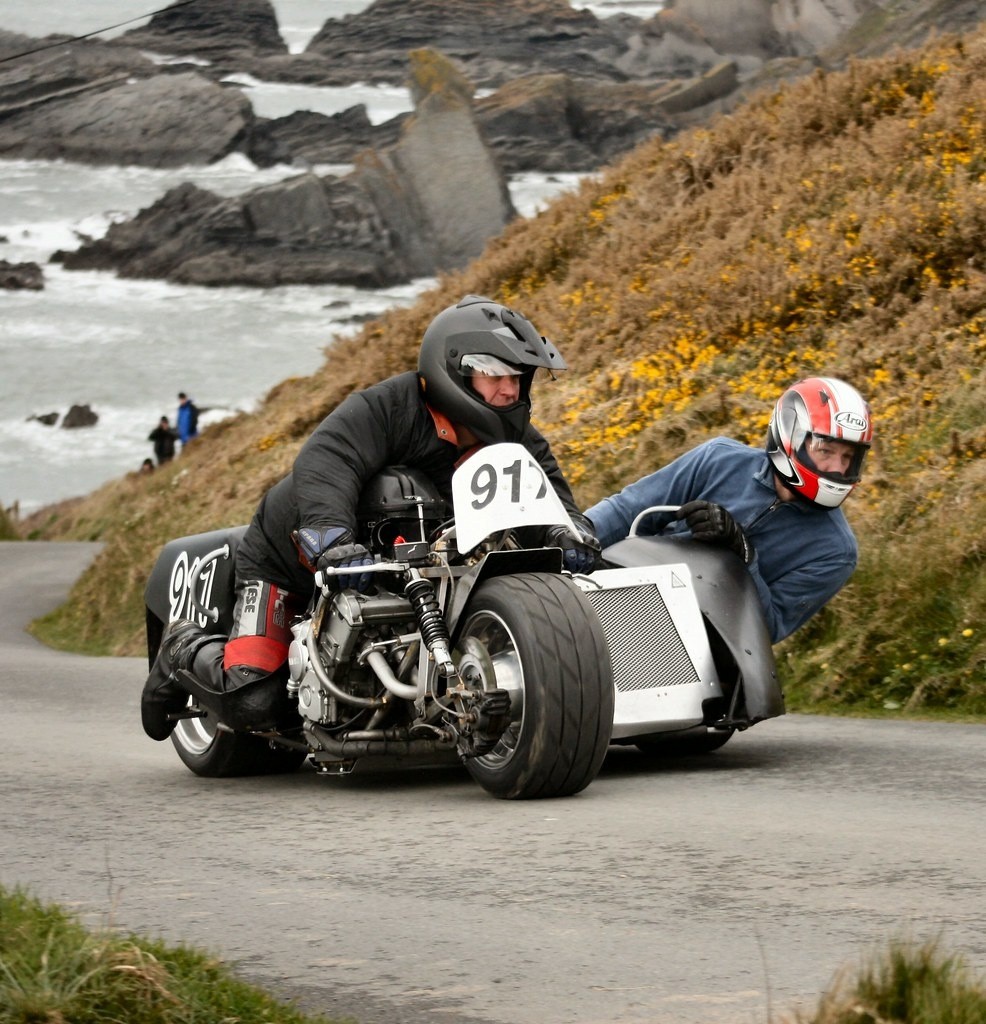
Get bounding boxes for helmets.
[767,375,871,508]
[416,295,569,444]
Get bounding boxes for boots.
[142,618,226,740]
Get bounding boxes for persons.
[141,458,154,473]
[147,416,178,467]
[174,392,199,452]
[138,292,602,743]
[581,374,874,647]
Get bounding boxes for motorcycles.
[146,437,791,798]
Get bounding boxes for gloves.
[674,499,756,567]
[314,541,376,592]
[548,525,604,575]
